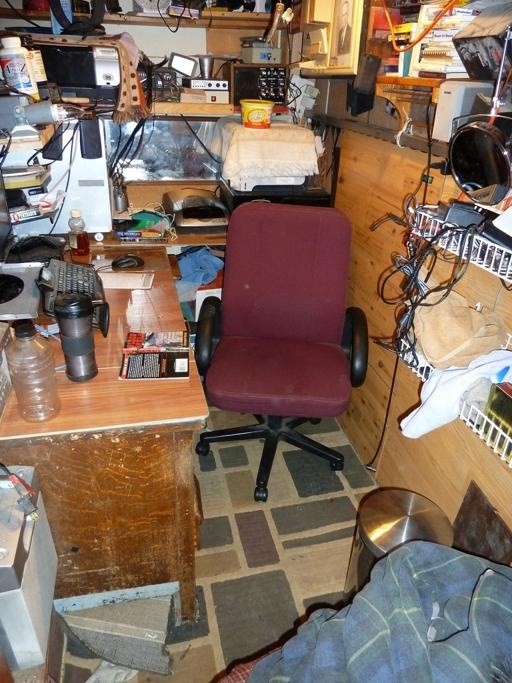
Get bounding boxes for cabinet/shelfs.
[0,7,299,120]
[396,203,512,473]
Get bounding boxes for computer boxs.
[0,117,114,236]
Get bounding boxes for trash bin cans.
[343,487,455,606]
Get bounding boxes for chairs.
[192,200,372,501]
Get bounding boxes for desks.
[0,222,226,628]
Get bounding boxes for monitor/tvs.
[0,164,24,305]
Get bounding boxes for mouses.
[111,253,145,269]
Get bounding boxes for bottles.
[68,219,91,264]
[6,321,61,424]
[0,34,47,95]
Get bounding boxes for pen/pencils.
[138,332,154,350]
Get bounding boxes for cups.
[54,292,110,382]
[199,58,214,78]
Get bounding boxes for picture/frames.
[299,0,371,79]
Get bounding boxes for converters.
[435,203,484,229]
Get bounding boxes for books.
[9,204,43,224]
[365,0,484,80]
[117,330,190,382]
[114,221,169,244]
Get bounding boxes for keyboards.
[39,257,106,318]
